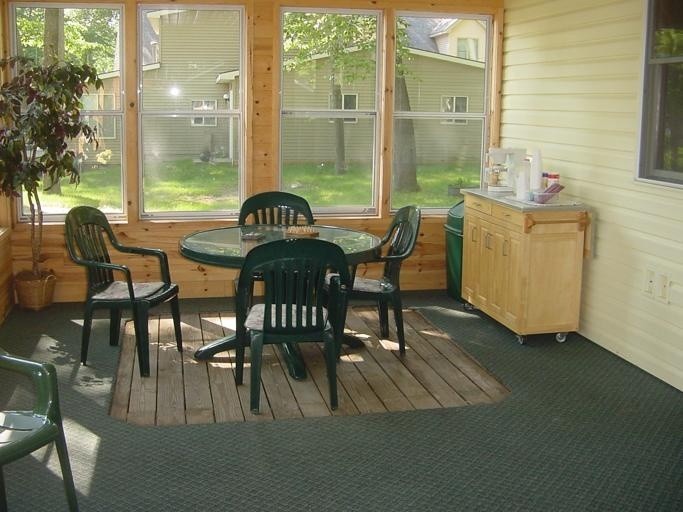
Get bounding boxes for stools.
[0,349,82,511]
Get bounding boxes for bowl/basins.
[533,192,559,206]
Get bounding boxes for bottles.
[541,172,547,190]
[514,158,532,200]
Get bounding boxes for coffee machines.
[484,148,526,192]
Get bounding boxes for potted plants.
[0,41,106,314]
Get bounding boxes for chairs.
[325,205,421,356]
[237,192,315,228]
[65,205,185,378]
[234,239,353,414]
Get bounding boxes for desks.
[177,227,381,365]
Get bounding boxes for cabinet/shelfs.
[461,187,591,346]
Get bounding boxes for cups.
[547,174,559,188]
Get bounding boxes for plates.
[240,233,264,240]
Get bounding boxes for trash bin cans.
[443,200,464,300]
